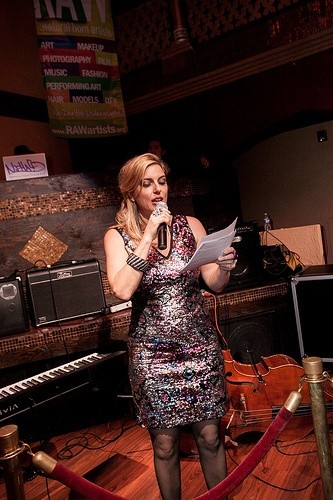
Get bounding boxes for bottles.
[264,213,271,231]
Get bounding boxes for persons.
[104,153,238,500]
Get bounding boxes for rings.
[153,209,163,216]
[233,253,237,258]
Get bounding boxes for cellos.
[176,347,333,449]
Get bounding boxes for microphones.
[155,201,168,248]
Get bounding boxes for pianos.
[0,347,138,445]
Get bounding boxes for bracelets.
[126,253,150,272]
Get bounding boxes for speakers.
[26,260,107,327]
[0,275,30,339]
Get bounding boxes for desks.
[0,281,290,384]
